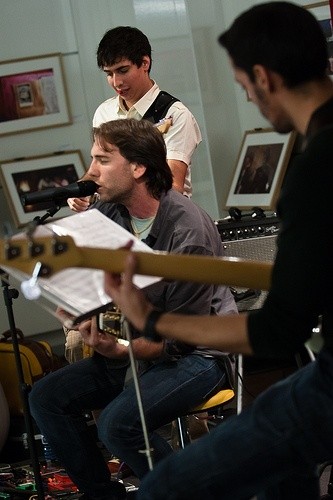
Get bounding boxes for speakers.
[213,214,282,311]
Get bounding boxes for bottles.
[42,435,59,468]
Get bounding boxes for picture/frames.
[300,0,333,83]
[0,51,73,137]
[0,148,88,228]
[220,127,297,210]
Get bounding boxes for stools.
[172,389,235,449]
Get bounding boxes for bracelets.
[143,307,169,343]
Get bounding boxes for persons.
[38,26,202,478]
[105,0,333,500]
[28,118,239,500]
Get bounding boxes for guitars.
[62,307,131,367]
[0,233,273,300]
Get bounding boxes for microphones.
[233,287,263,302]
[19,180,98,206]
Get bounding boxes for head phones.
[228,206,265,221]
